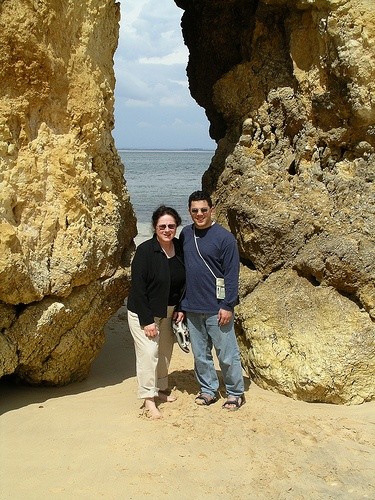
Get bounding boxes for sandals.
[195,393,219,405]
[222,394,245,410]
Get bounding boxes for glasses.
[192,207,207,213]
[159,224,177,230]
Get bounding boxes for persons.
[179,191,246,410]
[127,205,185,420]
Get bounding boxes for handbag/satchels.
[216,278,225,299]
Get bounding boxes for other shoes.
[172,320,190,354]
